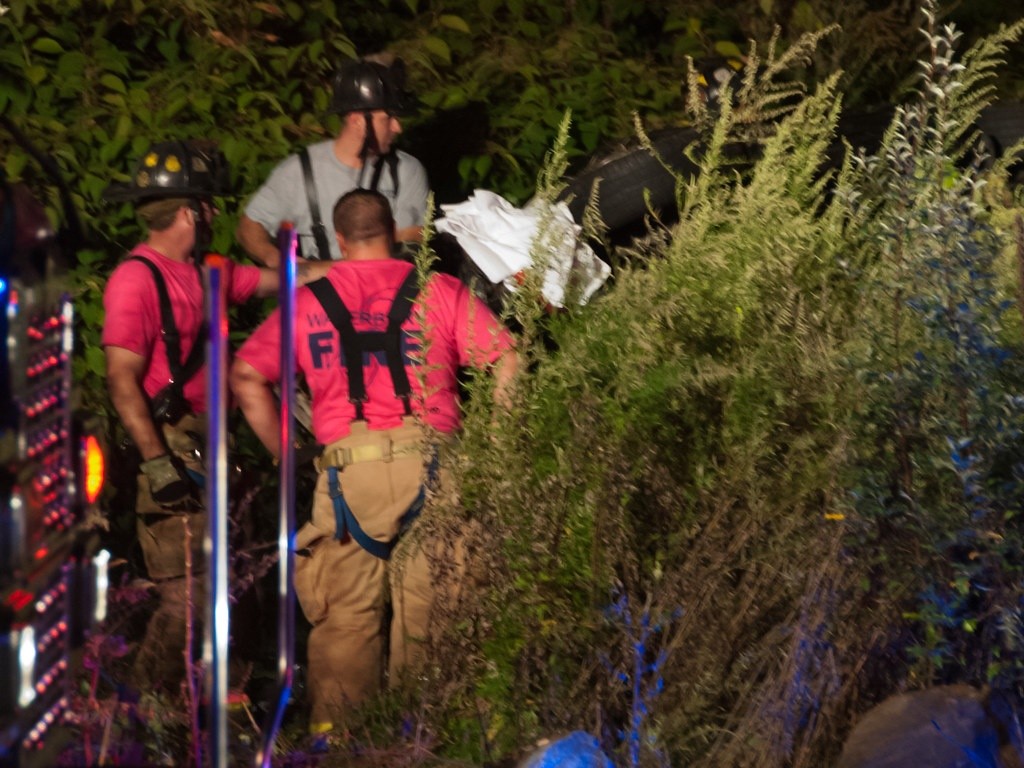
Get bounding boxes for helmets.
[336,60,411,112]
[127,140,232,200]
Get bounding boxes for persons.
[102,62,521,754]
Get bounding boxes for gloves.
[295,444,324,495]
[138,449,206,514]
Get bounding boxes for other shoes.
[179,680,251,713]
[312,727,350,746]
